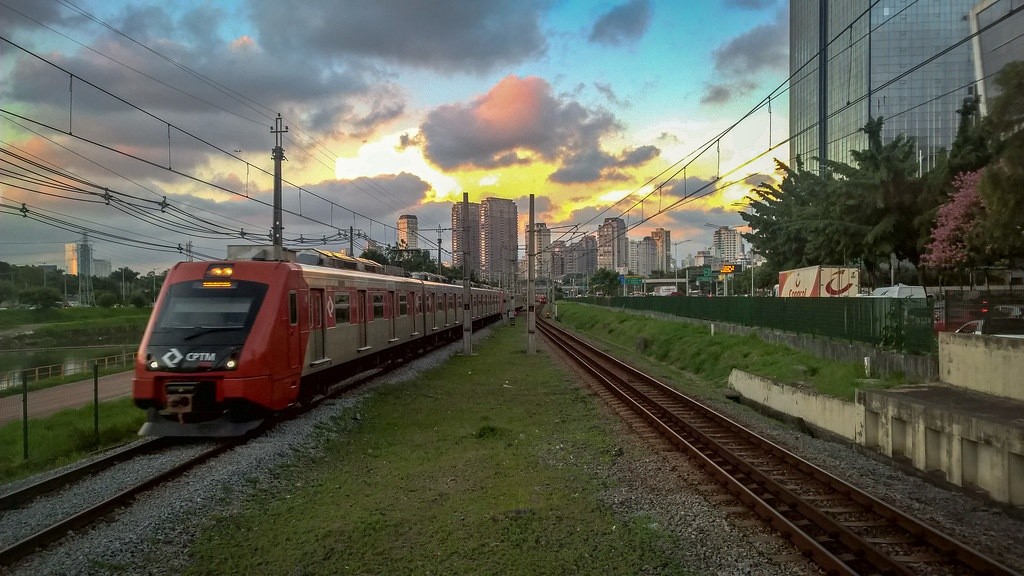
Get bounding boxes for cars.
[995,304,1024,318]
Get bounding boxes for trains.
[132,259,505,441]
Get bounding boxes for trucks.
[765,264,859,297]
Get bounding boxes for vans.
[869,286,927,299]
